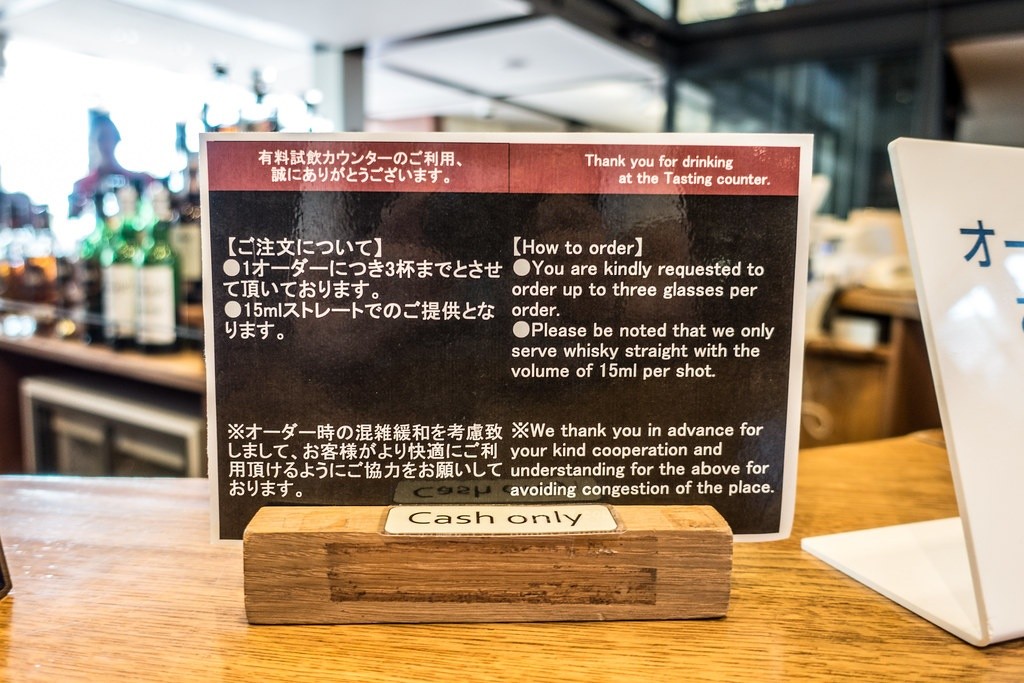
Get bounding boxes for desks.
[0,431,1024,683]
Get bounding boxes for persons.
[67,115,156,224]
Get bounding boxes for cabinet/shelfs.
[798,289,943,451]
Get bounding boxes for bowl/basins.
[832,318,879,351]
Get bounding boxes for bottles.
[134,189,184,352]
[102,189,138,350]
[81,191,113,341]
